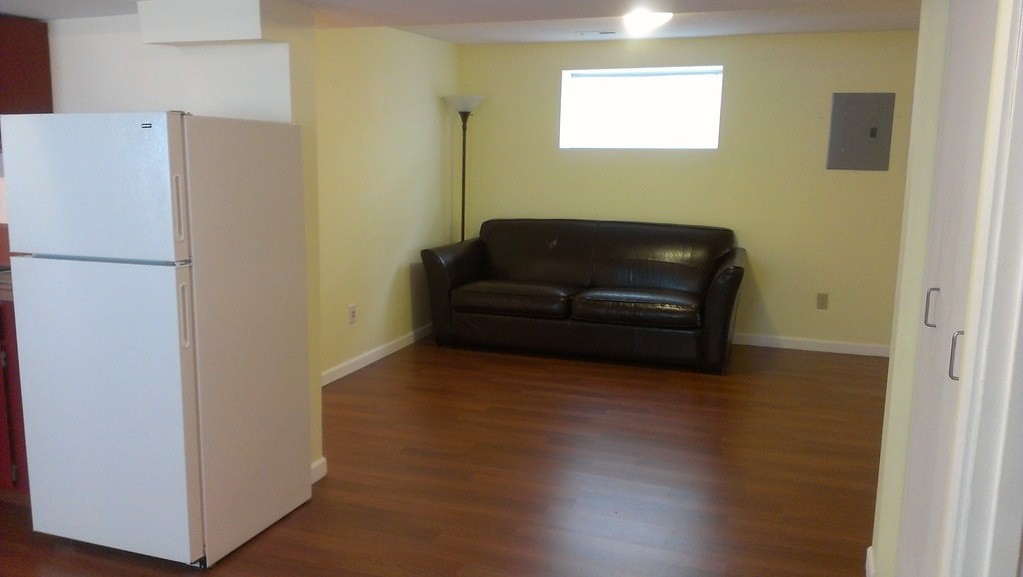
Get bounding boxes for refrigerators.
[0,110,312,570]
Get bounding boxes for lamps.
[442,95,483,241]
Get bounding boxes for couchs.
[421,218,748,374]
